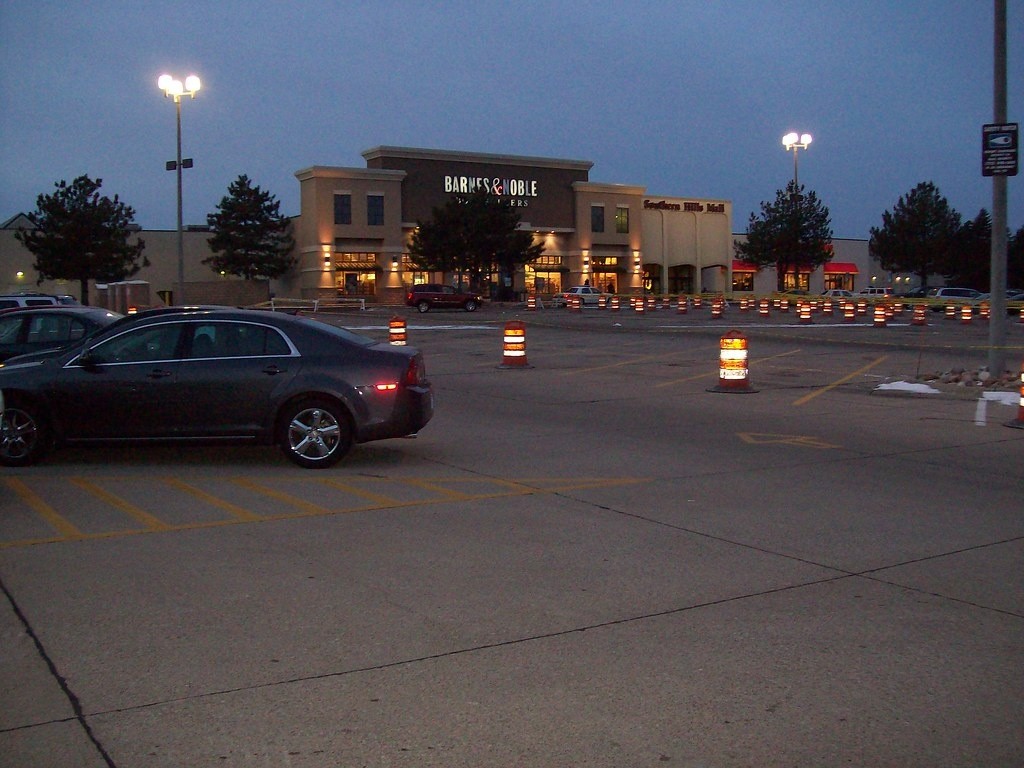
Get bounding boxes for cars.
[0,307,159,365]
[820,290,854,302]
[973,290,1024,316]
[556,285,614,307]
[777,290,820,306]
[0,305,434,471]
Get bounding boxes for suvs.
[0,294,65,328]
[926,288,982,313]
[852,287,897,299]
[59,296,84,310]
[405,284,484,312]
[896,286,944,311]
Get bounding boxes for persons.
[607,283,615,293]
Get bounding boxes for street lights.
[781,131,812,289]
[157,74,201,305]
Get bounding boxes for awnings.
[825,263,859,274]
[592,265,626,273]
[776,262,814,273]
[529,264,570,272]
[336,262,382,271]
[732,260,762,272]
[404,263,438,271]
